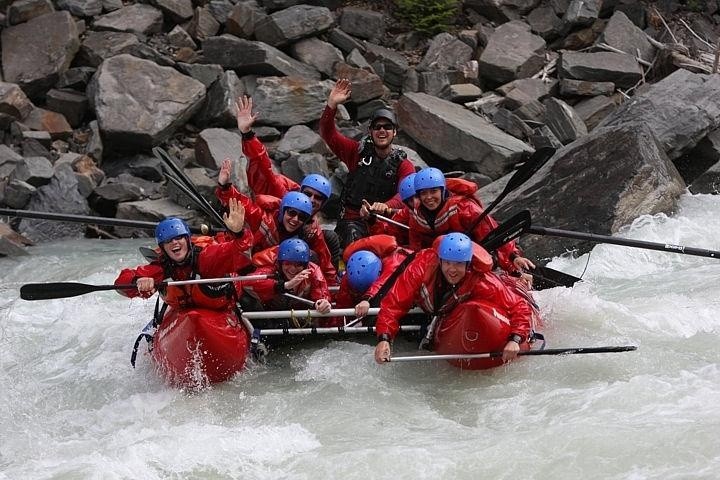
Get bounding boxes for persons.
[408,166,536,272]
[369,172,532,291]
[214,157,320,266]
[115,197,254,310]
[242,237,332,315]
[234,94,339,287]
[330,234,414,329]
[320,77,416,250]
[374,231,534,363]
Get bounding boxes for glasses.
[372,124,394,130]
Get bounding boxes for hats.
[371,109,397,127]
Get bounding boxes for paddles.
[384,345,636,361]
[152,146,228,230]
[462,146,555,235]
[20,274,280,300]
[368,210,581,287]
[479,210,532,251]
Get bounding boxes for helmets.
[437,232,473,263]
[155,217,191,245]
[346,250,382,291]
[398,167,446,202]
[278,173,333,266]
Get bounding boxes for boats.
[144,265,533,394]
